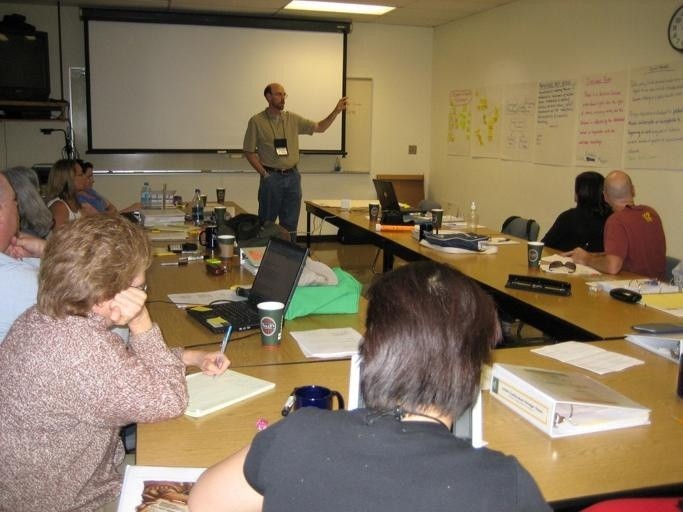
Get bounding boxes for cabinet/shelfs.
[373,173,425,210]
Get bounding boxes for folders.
[489,363,652,438]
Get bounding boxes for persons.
[539,171,613,253]
[241,83,349,239]
[560,171,666,282]
[1,159,232,512]
[187,261,553,512]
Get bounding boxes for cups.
[527,240,545,268]
[295,385,345,413]
[367,203,380,223]
[419,208,444,239]
[199,188,235,261]
[257,301,285,349]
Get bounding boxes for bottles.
[140,182,152,208]
[467,202,479,234]
[191,188,204,225]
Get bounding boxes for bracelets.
[263,171,267,176]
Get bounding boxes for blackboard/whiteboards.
[70,67,374,174]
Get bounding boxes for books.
[184,367,276,419]
[632,324,683,335]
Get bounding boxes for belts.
[263,164,297,175]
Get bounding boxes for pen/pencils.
[160,250,210,265]
[282,388,299,416]
[497,239,510,242]
[584,243,588,249]
[212,326,232,380]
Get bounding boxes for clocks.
[667,4,683,50]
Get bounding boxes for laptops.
[186,236,310,335]
[373,179,428,216]
